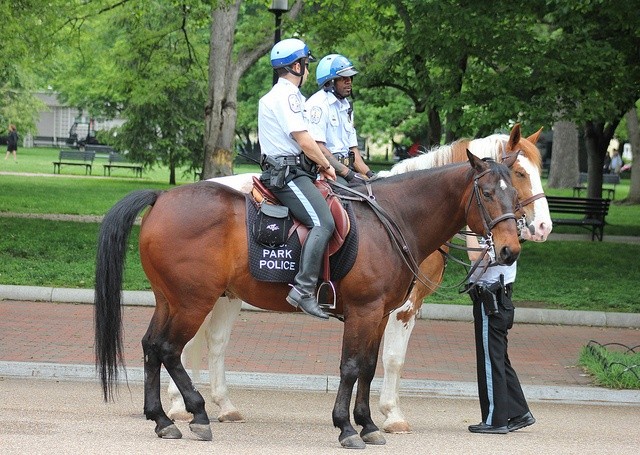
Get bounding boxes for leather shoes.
[508,412,535,432]
[468,422,508,434]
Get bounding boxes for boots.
[285,226,329,321]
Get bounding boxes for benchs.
[572,173,620,200]
[545,195,611,243]
[53,149,96,175]
[56,136,79,149]
[103,153,143,178]
[33,136,56,148]
[83,145,114,165]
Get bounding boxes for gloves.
[350,173,366,185]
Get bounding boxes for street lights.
[268,0,292,87]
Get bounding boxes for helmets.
[316,54,359,90]
[271,39,317,69]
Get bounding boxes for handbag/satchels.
[256,202,288,246]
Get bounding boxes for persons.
[602,151,612,174]
[609,148,622,182]
[465,222,536,434]
[4,124,19,163]
[257,38,338,320]
[305,53,377,186]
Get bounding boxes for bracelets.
[323,163,331,171]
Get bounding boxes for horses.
[92,147,522,449]
[165,122,552,437]
